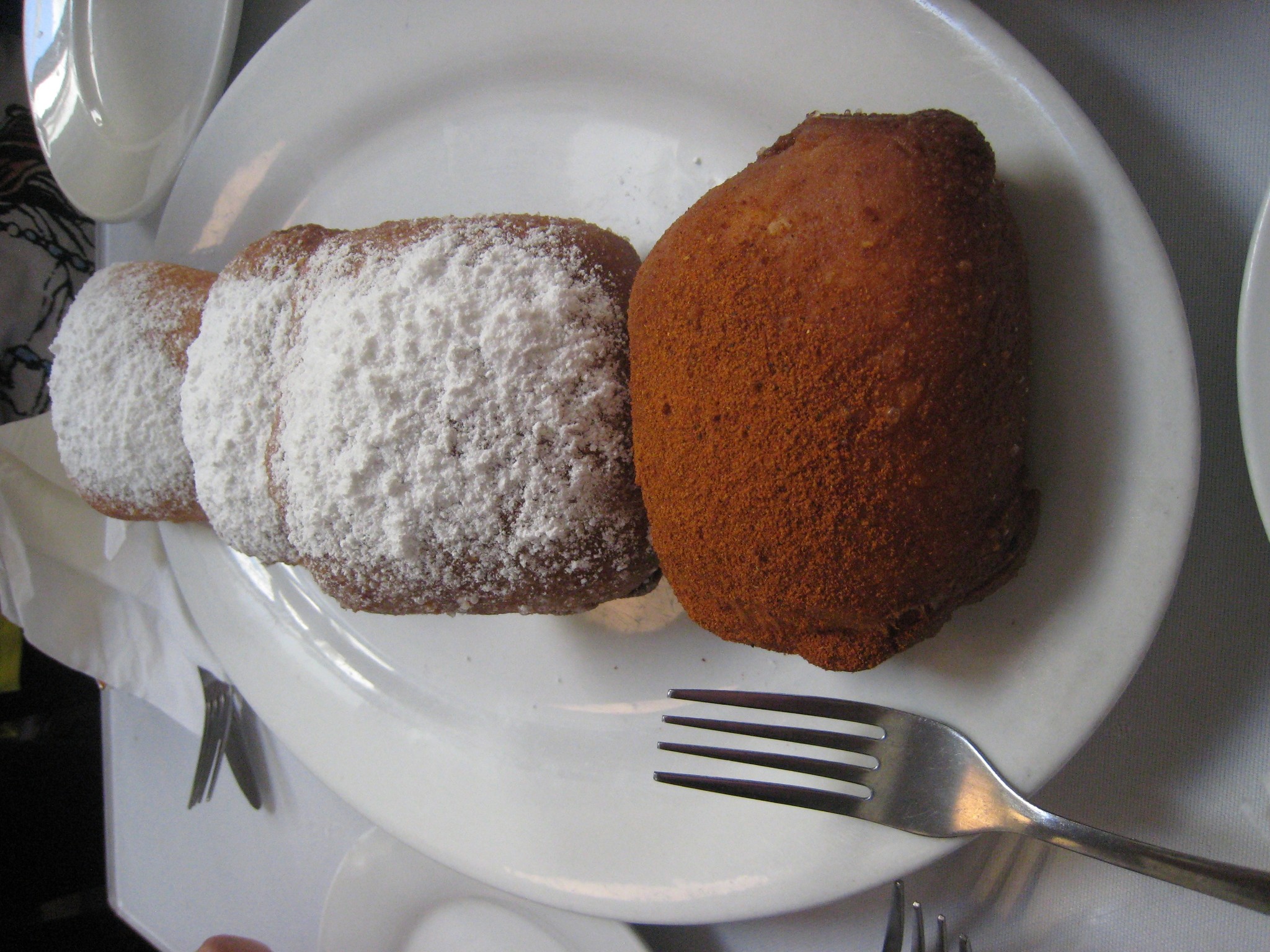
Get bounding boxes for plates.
[317,827,651,952]
[1236,186,1269,544]
[21,0,240,224]
[153,2,1205,926]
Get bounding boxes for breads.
[282,209,661,626]
[42,260,216,526]
[607,108,1040,678]
[172,224,346,571]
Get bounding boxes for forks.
[186,663,229,808]
[881,878,974,952]
[654,685,1270,915]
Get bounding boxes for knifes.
[224,700,260,811]
